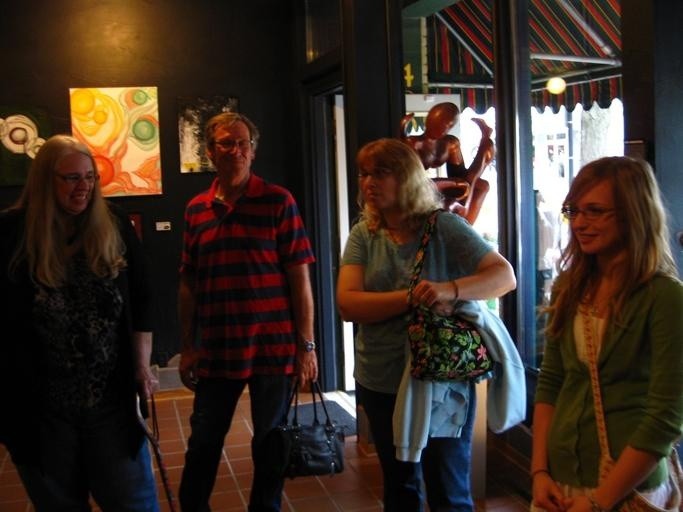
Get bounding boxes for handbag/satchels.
[598,449,683,512]
[405,308,493,381]
[274,419,343,477]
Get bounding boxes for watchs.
[587,493,610,512]
[297,340,315,352]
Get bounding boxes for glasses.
[56,170,96,183]
[562,205,618,220]
[356,169,401,180]
[212,140,255,151]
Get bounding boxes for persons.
[397,102,494,226]
[336,139,517,512]
[534,201,555,357]
[176,111,316,512]
[0,135,161,512]
[529,157,683,512]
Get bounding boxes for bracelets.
[530,470,552,484]
[448,279,459,306]
